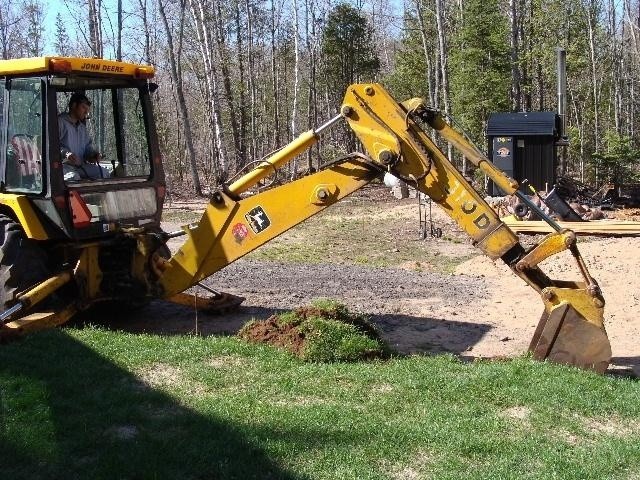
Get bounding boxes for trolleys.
[416,185,442,240]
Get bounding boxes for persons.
[54,93,113,184]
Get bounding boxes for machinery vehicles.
[1,54,614,381]
